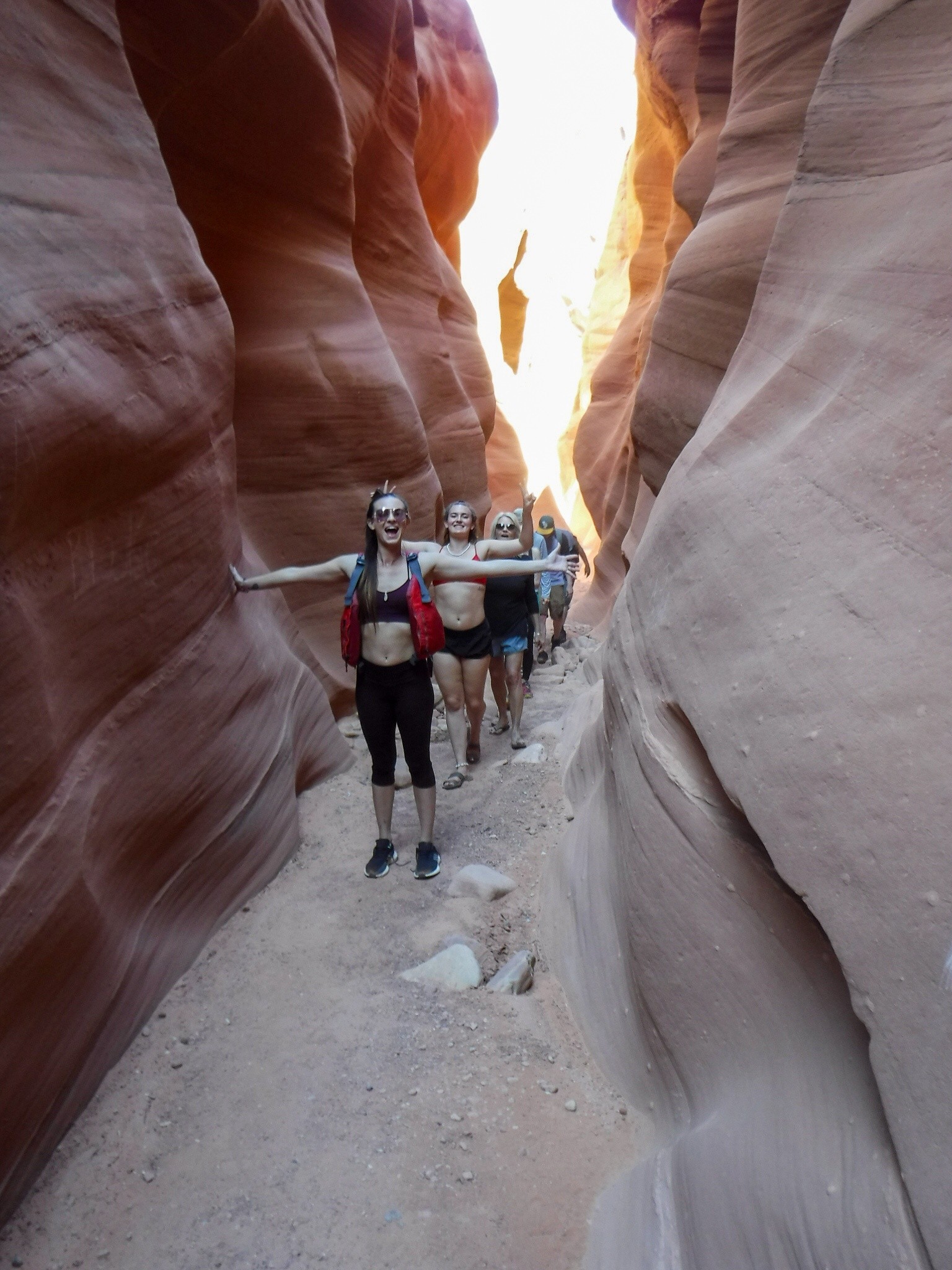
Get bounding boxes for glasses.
[496,524,515,530]
[371,508,406,521]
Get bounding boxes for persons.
[230,493,582,881]
[370,477,537,789]
[483,508,590,749]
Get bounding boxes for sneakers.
[411,841,440,878]
[364,839,411,878]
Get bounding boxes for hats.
[538,515,554,535]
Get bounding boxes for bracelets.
[242,578,248,592]
[535,632,541,636]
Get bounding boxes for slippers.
[443,772,468,789]
[489,725,509,734]
[467,742,480,763]
[511,737,526,749]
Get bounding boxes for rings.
[564,568,568,573]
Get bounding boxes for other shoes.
[523,681,532,698]
[560,630,566,643]
[551,634,560,652]
[537,651,548,664]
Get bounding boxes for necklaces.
[447,542,470,556]
